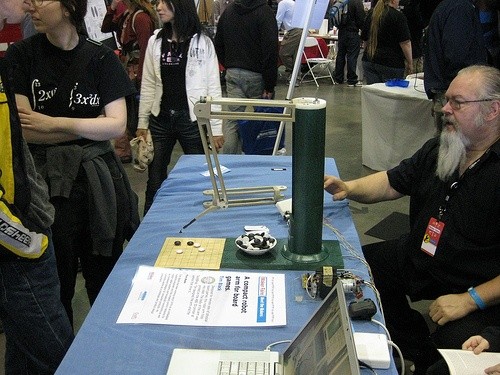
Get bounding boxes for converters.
[354,332,390,369]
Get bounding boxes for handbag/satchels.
[236,98,285,155]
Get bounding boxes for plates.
[235,233,277,256]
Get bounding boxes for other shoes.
[286,72,302,81]
[347,80,364,87]
[121,155,133,163]
[287,81,299,86]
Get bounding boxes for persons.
[362,0,415,84]
[136,0,225,217]
[423,0,495,137]
[215,0,281,155]
[329,0,367,87]
[0,1,163,375]
[325,64,500,375]
[275,0,303,87]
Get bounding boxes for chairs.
[300,37,335,88]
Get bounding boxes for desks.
[360,76,437,171]
[54,156,399,375]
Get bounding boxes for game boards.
[152,237,227,271]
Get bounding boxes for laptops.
[167,280,361,375]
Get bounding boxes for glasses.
[24,0,59,7]
[439,94,492,110]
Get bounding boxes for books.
[437,349,500,375]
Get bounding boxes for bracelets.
[468,287,486,309]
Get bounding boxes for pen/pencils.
[272,168,287,171]
